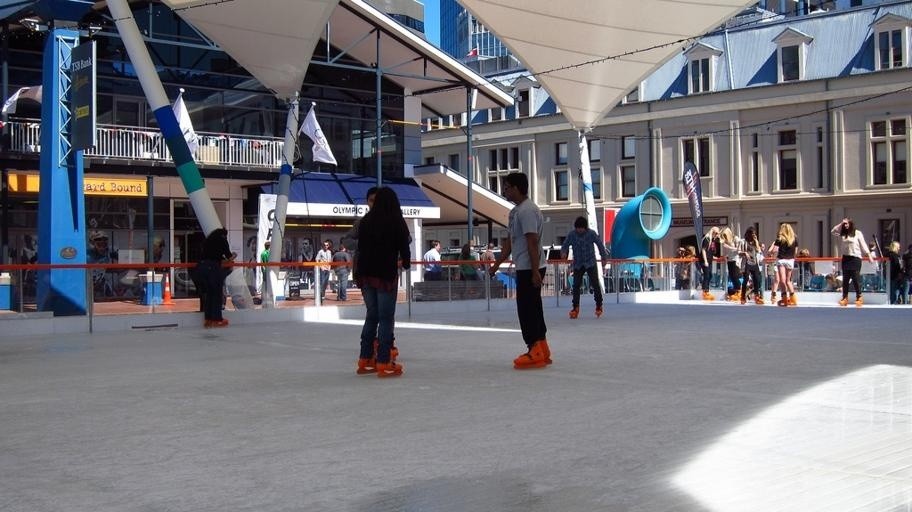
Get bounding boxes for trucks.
[438,244,516,274]
[540,244,573,284]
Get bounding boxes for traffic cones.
[162,272,178,308]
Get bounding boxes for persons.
[145,236,170,272]
[261,242,272,279]
[561,216,607,315]
[86,231,118,297]
[298,237,316,271]
[317,242,498,303]
[674,216,912,307]
[23,235,38,282]
[199,229,237,326]
[244,236,257,286]
[489,173,550,364]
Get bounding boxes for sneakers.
[356,355,376,368]
[202,317,228,327]
[700,289,865,307]
[567,304,605,318]
[537,338,552,359]
[375,361,403,372]
[513,343,545,365]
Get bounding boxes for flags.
[173,96,199,161]
[301,108,338,166]
[466,48,477,57]
